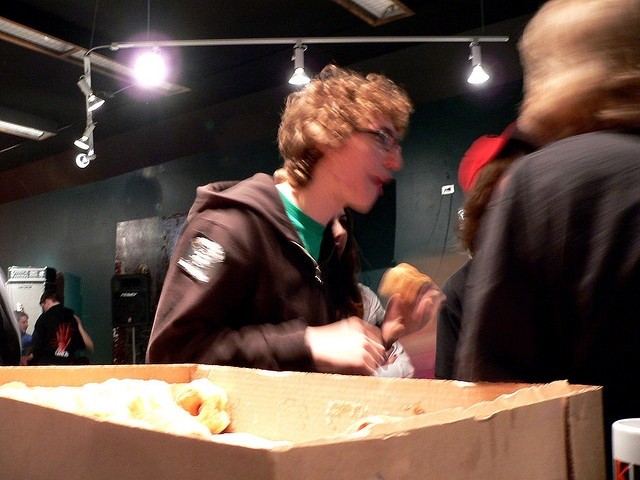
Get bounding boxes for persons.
[437,113,537,380]
[434,0,640,480]
[145,64,445,377]
[31,291,94,365]
[14,310,32,356]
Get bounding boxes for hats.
[458,123,517,194]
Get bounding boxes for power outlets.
[441,184,454,196]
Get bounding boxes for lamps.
[466,45,491,84]
[77,74,105,112]
[74,123,96,151]
[76,153,96,168]
[288,45,313,85]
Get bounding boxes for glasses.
[353,128,403,152]
